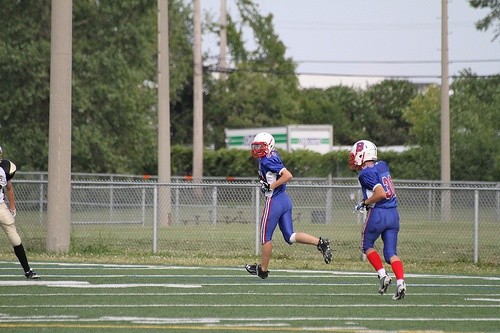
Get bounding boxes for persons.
[0,146,41,279]
[245,132,332,279]
[348,140,406,300]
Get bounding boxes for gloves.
[353,199,366,214]
[10,209,16,217]
[258,176,271,192]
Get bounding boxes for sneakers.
[244,263,268,280]
[24,269,41,281]
[376,270,393,293]
[391,281,406,301]
[316,236,333,265]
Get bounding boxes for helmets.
[251,132,275,159]
[348,139,378,171]
